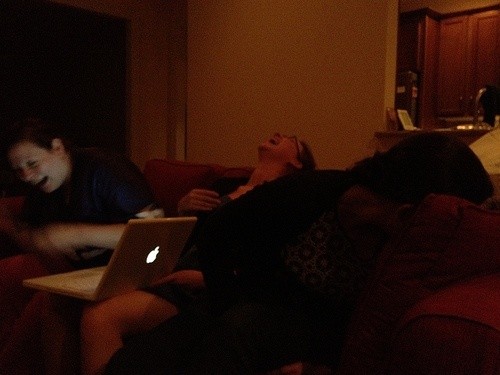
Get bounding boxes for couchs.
[0,158,500,375]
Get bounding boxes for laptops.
[22,216,197,302]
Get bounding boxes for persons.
[103,132,500,375]
[0,115,165,374]
[39,131,317,375]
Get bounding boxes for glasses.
[287,135,300,160]
